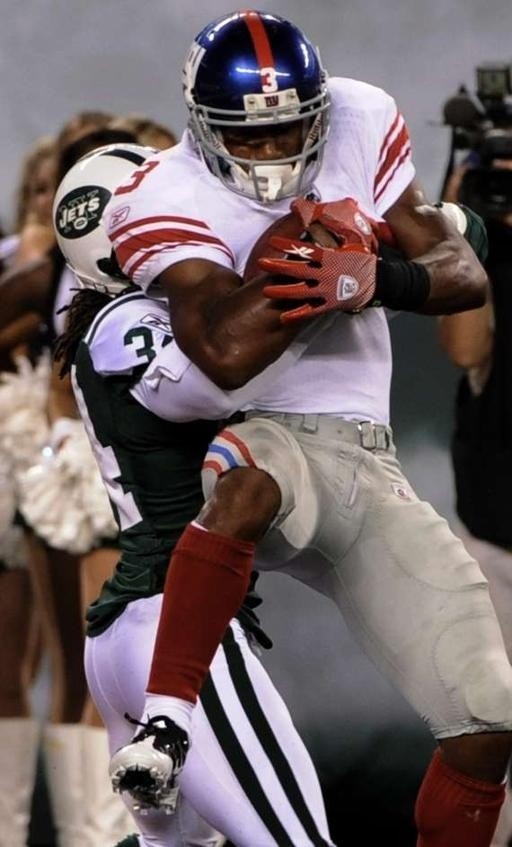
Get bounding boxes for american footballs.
[244,212,315,287]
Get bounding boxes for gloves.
[259,194,380,326]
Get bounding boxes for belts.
[271,411,390,451]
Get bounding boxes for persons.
[1,110,332,844]
[99,9,511,847]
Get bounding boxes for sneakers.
[109,718,187,815]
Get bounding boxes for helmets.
[50,144,165,299]
[180,10,331,202]
